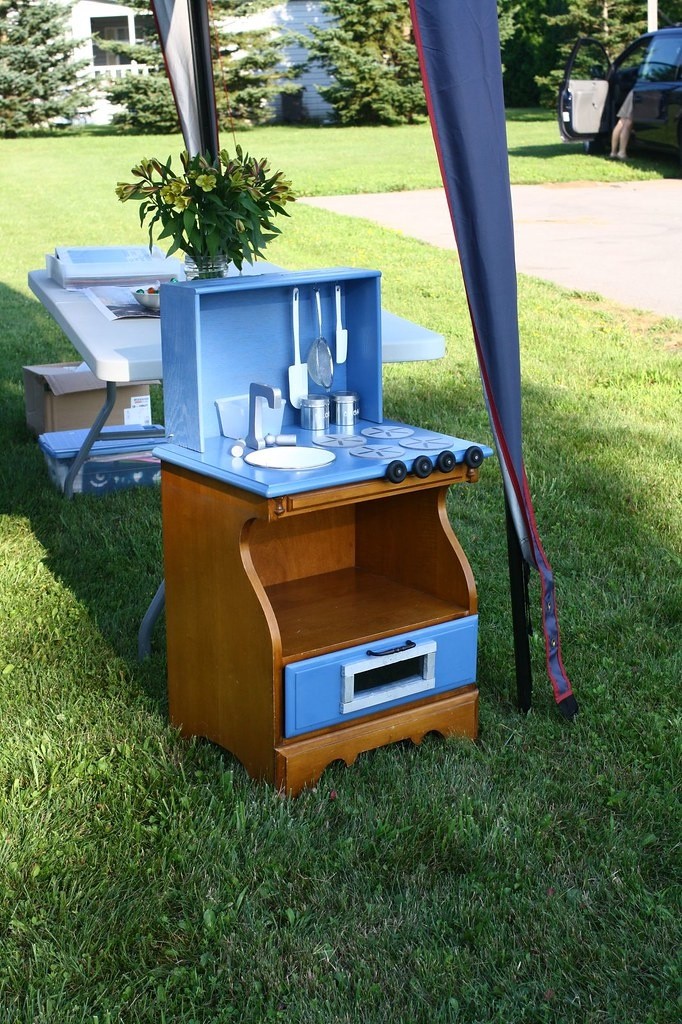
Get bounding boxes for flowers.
[115,144,298,280]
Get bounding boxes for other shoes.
[610,153,617,158]
[617,153,628,159]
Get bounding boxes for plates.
[131,290,159,307]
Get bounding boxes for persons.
[610,88,633,159]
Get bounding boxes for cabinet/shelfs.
[162,458,479,803]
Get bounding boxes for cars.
[557,27,682,160]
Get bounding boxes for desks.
[27,256,445,658]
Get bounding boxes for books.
[54,244,178,289]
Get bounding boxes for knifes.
[335,286,348,364]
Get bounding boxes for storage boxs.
[23,362,153,443]
[215,393,287,439]
[38,424,165,496]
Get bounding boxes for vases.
[184,255,228,279]
